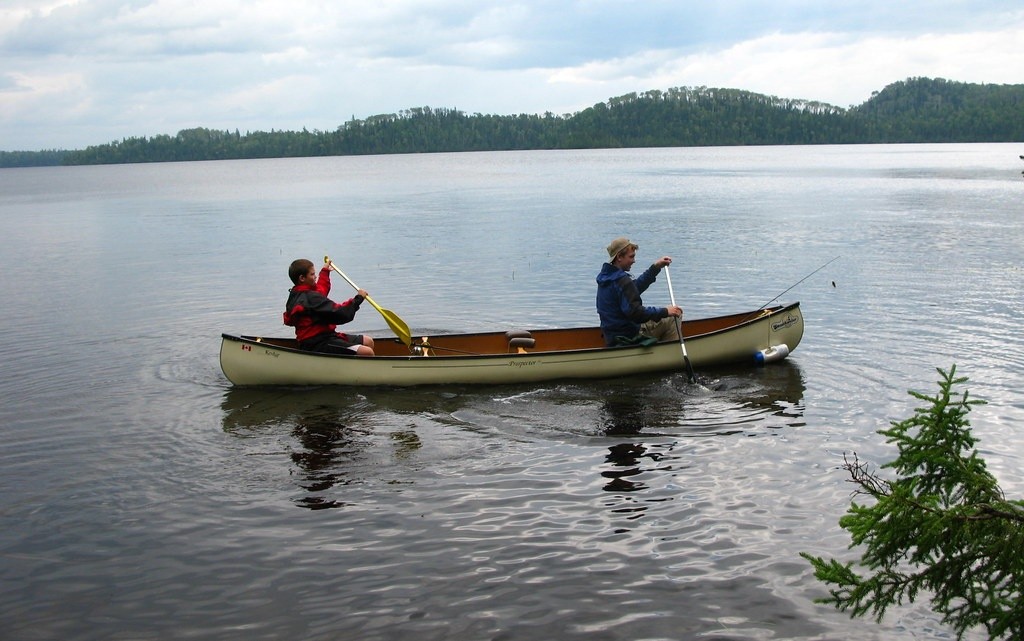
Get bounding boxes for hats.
[606,237,631,264]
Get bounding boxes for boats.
[218,299,806,388]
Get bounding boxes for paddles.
[325,257,411,348]
[664,264,697,385]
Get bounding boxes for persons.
[283,258,375,356]
[596,236,683,347]
